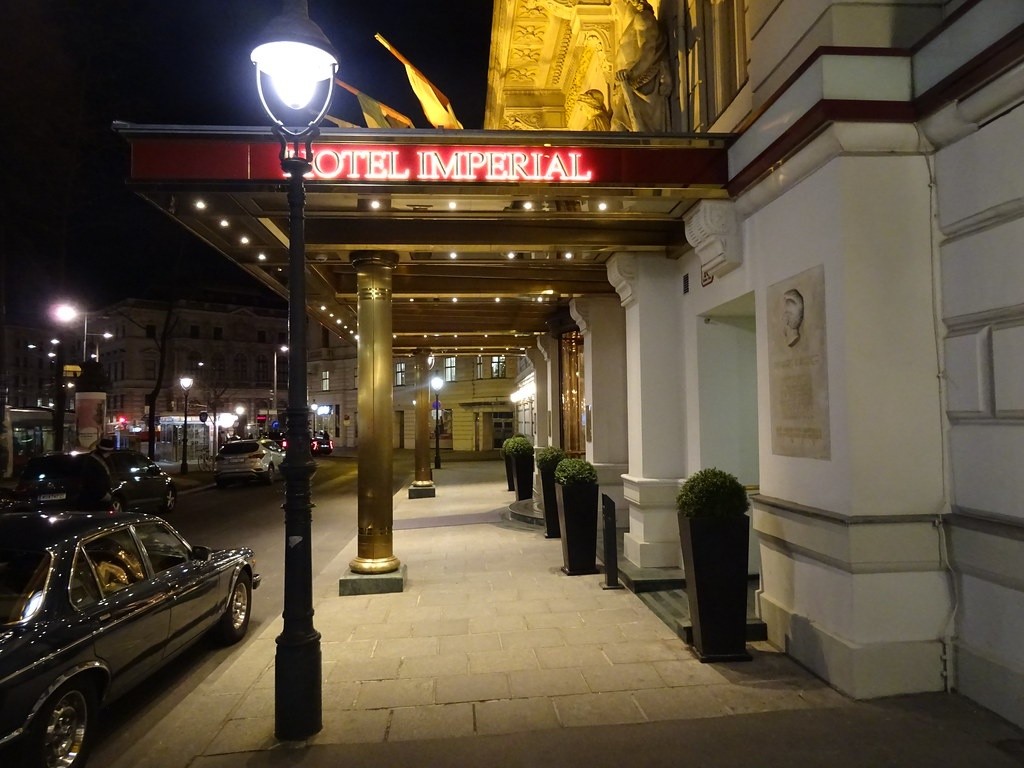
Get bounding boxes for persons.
[610,0,663,132]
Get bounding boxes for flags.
[407,64,463,131]
[356,93,415,129]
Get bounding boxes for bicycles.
[194,448,214,472]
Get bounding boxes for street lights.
[248,1,342,740]
[82,312,114,362]
[430,376,446,469]
[49,301,79,452]
[179,369,193,474]
[310,403,319,437]
[274,346,290,409]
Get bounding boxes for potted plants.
[554,459,599,576]
[502,433,526,492]
[674,467,754,664]
[508,436,534,502]
[536,445,568,539]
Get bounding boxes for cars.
[0,450,178,519]
[268,429,334,459]
[0,513,262,768]
[213,439,286,485]
